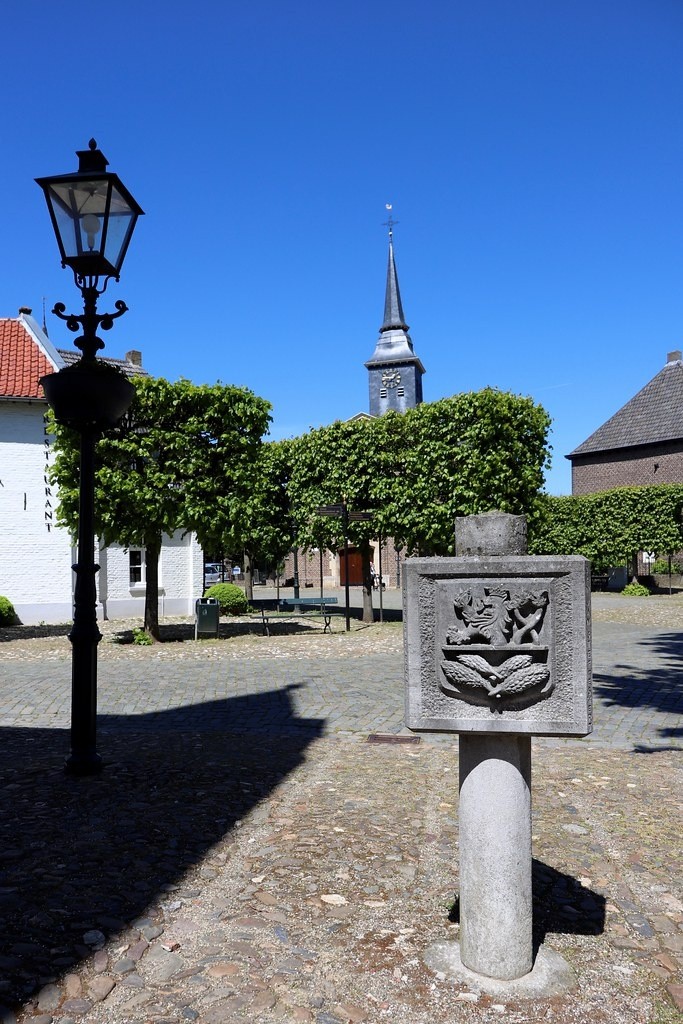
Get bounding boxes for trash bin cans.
[608,567,628,589]
[194,598,220,640]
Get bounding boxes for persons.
[370,562,376,585]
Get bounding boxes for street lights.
[33,136,145,784]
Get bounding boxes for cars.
[204,567,221,588]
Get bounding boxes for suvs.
[205,563,230,582]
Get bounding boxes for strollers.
[373,574,385,592]
